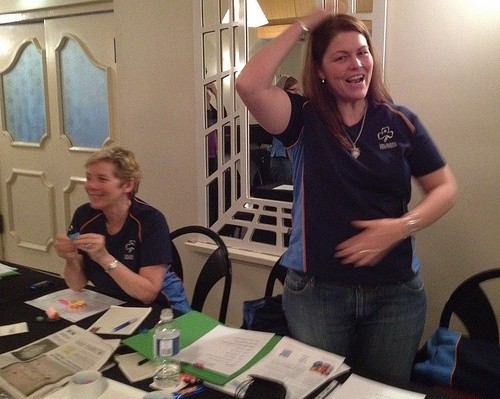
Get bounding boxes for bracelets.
[401,213,418,237]
[295,18,309,42]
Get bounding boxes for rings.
[87,244,93,249]
[359,250,367,258]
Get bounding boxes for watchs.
[105,259,118,274]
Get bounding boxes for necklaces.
[335,98,367,159]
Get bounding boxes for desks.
[0,256,455,399]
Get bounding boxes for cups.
[69,370,102,399]
[141,391,175,399]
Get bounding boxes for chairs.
[437,266,500,398]
[169,225,233,324]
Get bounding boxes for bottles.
[152,308,180,389]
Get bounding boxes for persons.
[234,9,457,391]
[269,75,300,183]
[52,145,173,309]
[18,339,57,359]
[206,68,231,219]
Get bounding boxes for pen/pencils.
[113,318,139,333]
[137,358,149,366]
